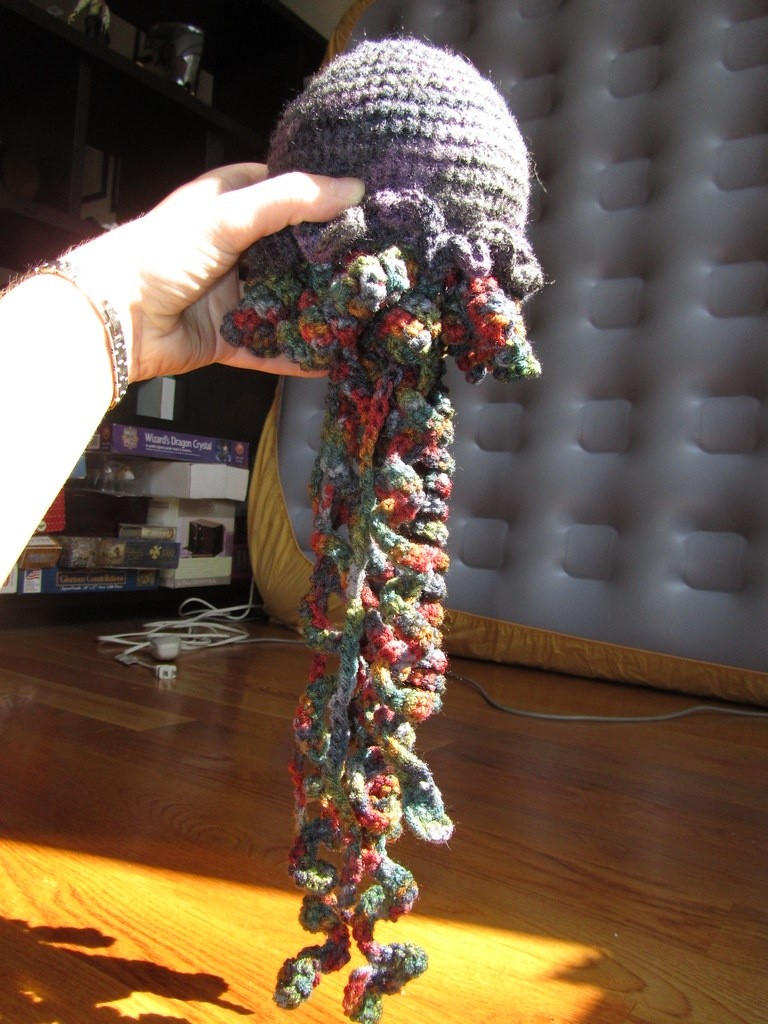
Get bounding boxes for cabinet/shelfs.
[0,0,326,627]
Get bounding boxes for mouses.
[150,636,181,661]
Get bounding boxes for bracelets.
[34,260,129,409]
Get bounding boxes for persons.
[0,163,366,590]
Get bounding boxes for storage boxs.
[135,459,250,502]
[99,423,249,468]
[17,535,63,567]
[18,567,159,595]
[57,535,180,571]
[147,496,236,589]
[0,563,18,594]
